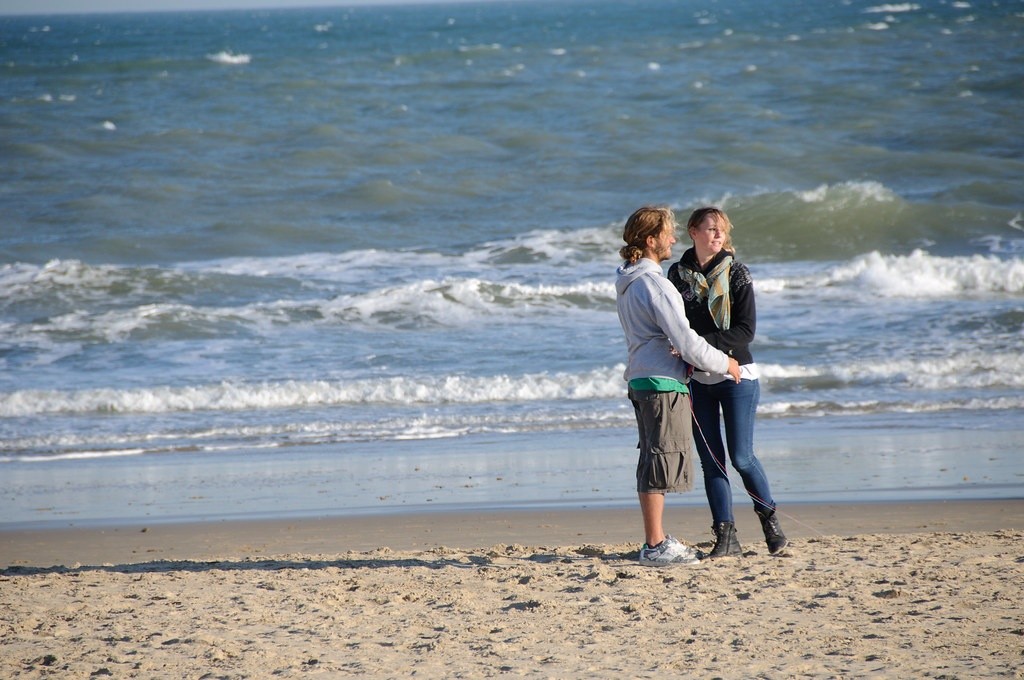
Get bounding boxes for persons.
[668,207,789,562]
[616,204,741,567]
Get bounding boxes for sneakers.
[639,534,703,566]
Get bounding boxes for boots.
[754,503,788,555]
[699,519,744,560]
